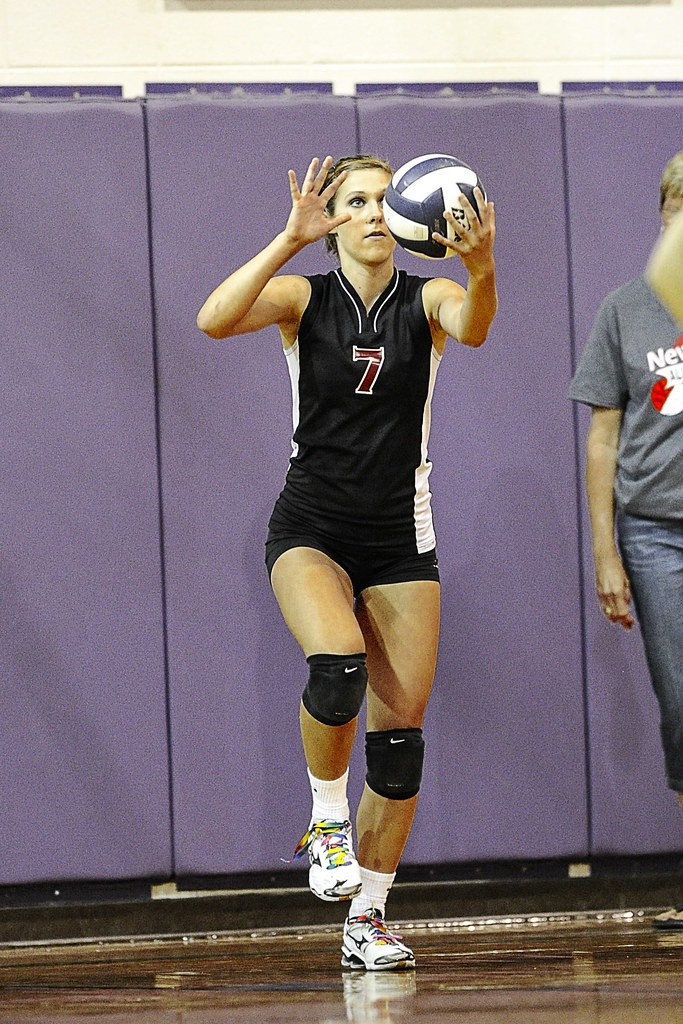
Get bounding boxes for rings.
[603,606,610,616]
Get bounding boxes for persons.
[570,148,683,807]
[196,154,499,967]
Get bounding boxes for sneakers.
[340,907,415,970]
[289,818,362,901]
[344,972,417,1019]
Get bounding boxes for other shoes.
[651,903,683,928]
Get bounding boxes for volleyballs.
[381,152,490,262]
